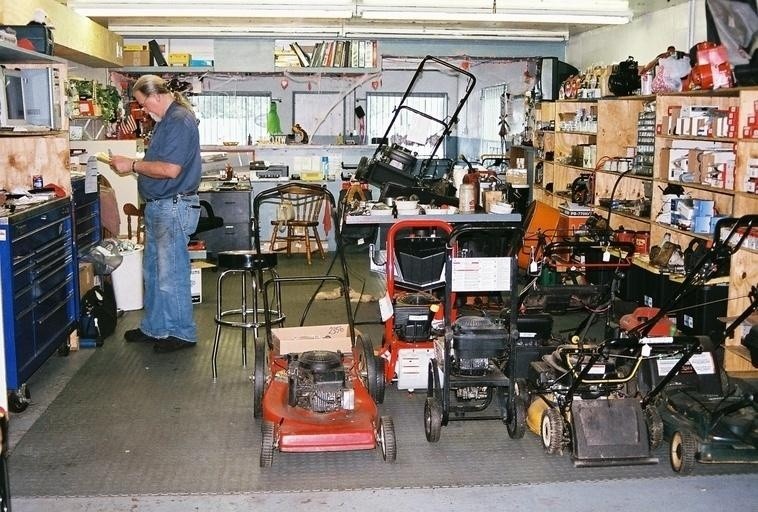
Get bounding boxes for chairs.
[190,200,224,236]
[269,184,327,265]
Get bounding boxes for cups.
[386,197,397,206]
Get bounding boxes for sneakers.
[155,336,196,353]
[124,327,157,343]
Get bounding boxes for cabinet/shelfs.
[533,86,758,380]
[0,195,76,412]
[193,189,251,272]
[0,40,67,137]
[70,196,103,322]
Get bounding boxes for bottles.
[459,174,475,213]
[564,115,597,135]
[33,174,44,191]
[322,156,329,175]
[602,145,635,173]
[392,201,399,220]
[641,72,653,95]
[576,143,596,168]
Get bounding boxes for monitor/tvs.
[541,57,579,102]
[0,68,28,132]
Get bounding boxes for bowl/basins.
[489,201,514,214]
[393,200,420,209]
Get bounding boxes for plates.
[223,141,240,146]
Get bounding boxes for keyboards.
[12,125,51,131]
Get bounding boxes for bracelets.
[131,159,136,173]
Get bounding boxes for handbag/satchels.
[81,286,116,338]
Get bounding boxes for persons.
[108,73,203,353]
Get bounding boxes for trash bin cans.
[111,244,145,312]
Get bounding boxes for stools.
[211,250,287,383]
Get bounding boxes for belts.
[147,193,194,202]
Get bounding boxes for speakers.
[355,106,365,118]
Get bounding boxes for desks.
[346,200,522,265]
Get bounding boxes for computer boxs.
[21,67,63,131]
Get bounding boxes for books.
[273,36,377,68]
[83,152,134,195]
